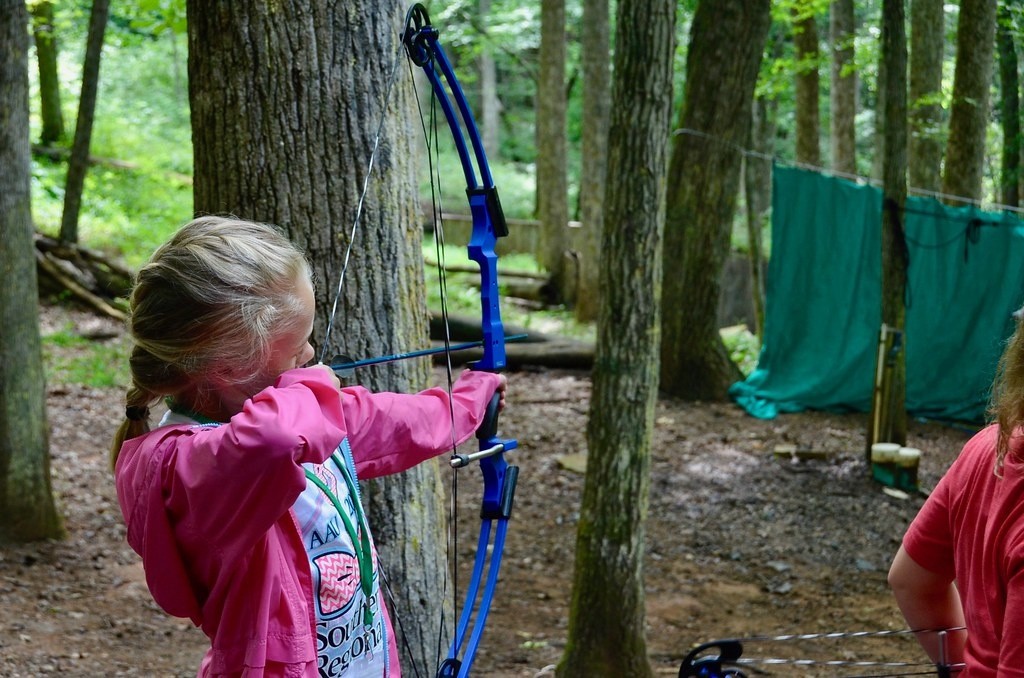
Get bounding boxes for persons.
[888,306,1024,678]
[111,215,509,678]
